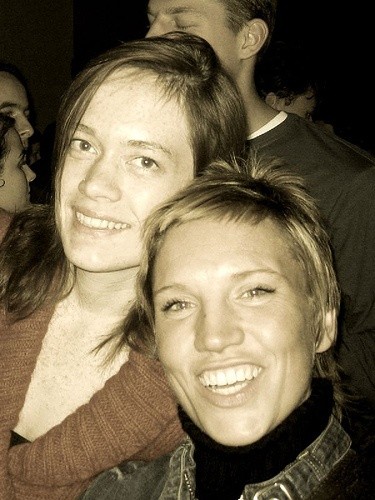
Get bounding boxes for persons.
[0,114,36,213]
[256,70,325,121]
[0,70,34,161]
[85,161,375,500]
[0,33,249,500]
[146,0,375,398]
